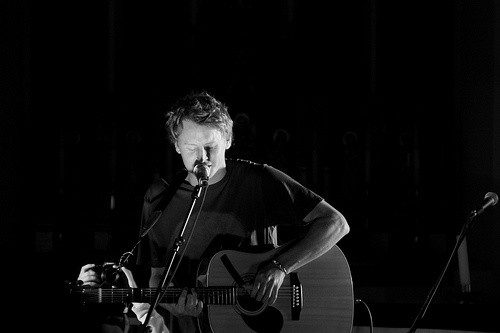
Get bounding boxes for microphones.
[468,192,498,218]
[192,160,210,187]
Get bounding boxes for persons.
[78,92,350,333]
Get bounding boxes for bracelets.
[270,259,289,276]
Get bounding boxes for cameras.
[85,265,128,289]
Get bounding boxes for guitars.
[53,236,354,333]
